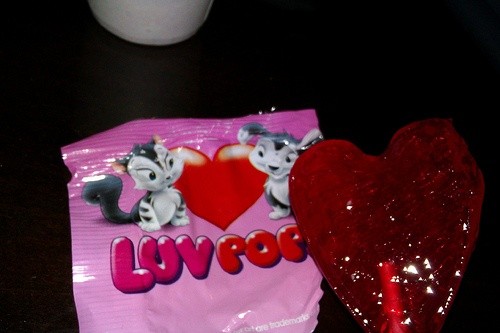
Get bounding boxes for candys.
[287,117,484,333]
